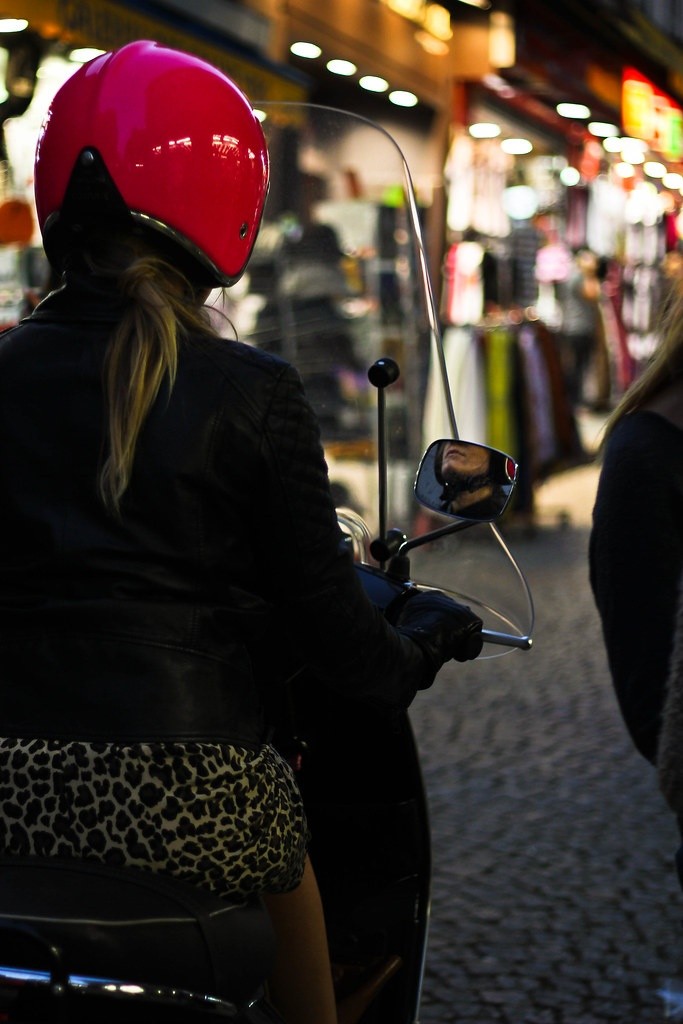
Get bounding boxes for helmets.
[34,40,270,288]
[434,441,514,486]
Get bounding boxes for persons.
[558,234,683,827]
[0,39,481,1023]
[434,435,515,520]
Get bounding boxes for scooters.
[0,101,537,1023]
